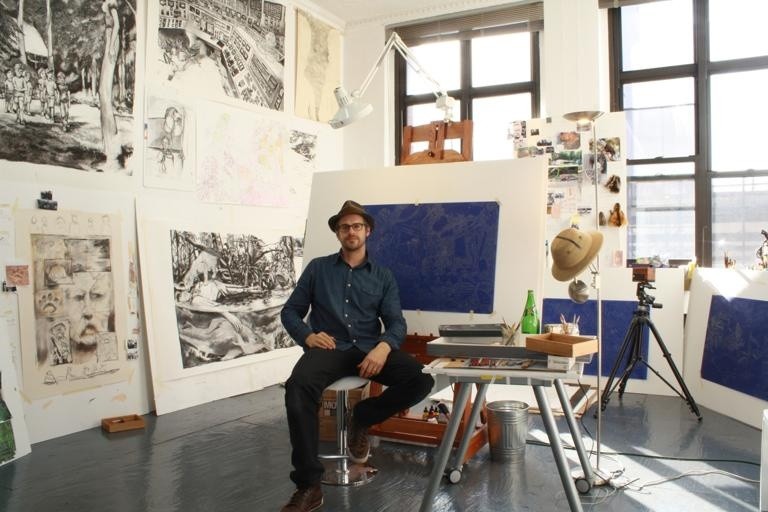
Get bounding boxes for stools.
[316,379,381,487]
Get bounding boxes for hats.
[327,200,375,232]
[550,227,604,282]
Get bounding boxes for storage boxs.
[318,378,371,446]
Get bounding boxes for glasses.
[336,222,368,233]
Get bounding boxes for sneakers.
[345,410,370,464]
[279,481,324,512]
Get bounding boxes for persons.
[2,62,72,133]
[96,1,122,145]
[600,138,616,157]
[59,273,115,363]
[609,202,626,227]
[279,198,436,512]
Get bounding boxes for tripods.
[593,284,704,422]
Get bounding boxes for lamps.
[561,109,608,486]
[329,31,456,129]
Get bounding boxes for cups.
[724,249,737,268]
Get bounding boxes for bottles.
[521,290,540,334]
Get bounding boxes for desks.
[419,373,596,511]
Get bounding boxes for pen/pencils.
[560,312,581,334]
[499,314,521,347]
